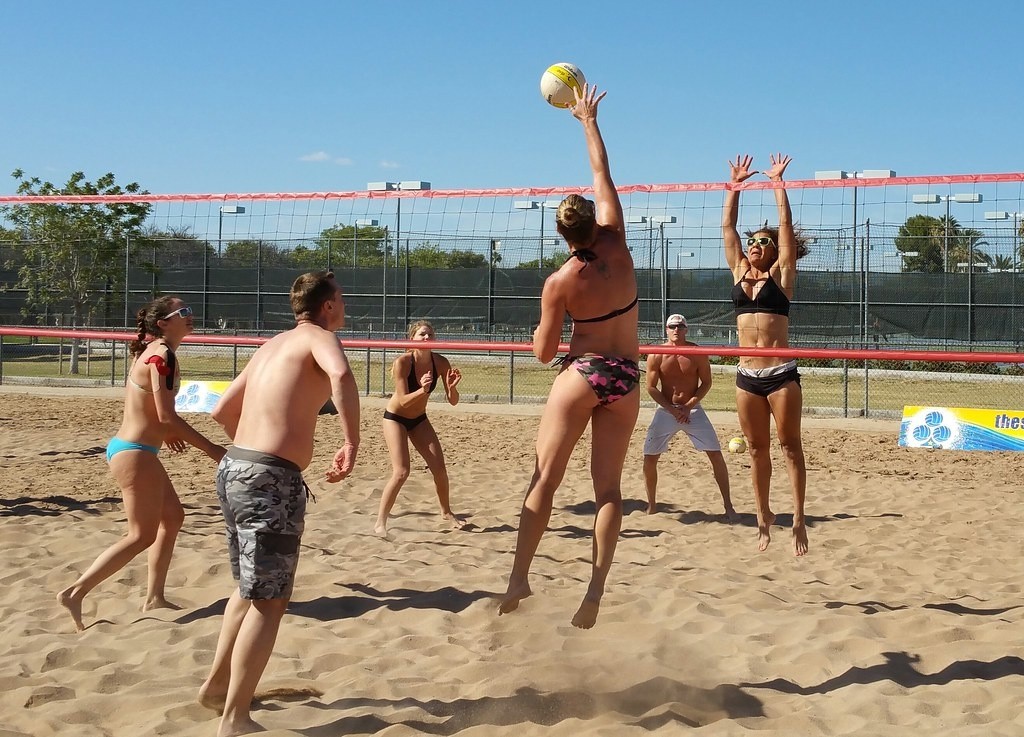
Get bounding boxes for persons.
[721,151,809,556]
[373,319,468,538]
[56,294,228,630]
[643,313,742,525]
[499,81,641,630]
[197,270,361,736]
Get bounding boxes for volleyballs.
[540,62,588,109]
[728,437,746,453]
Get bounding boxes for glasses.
[159,307,193,319]
[746,237,776,249]
[667,324,687,329]
